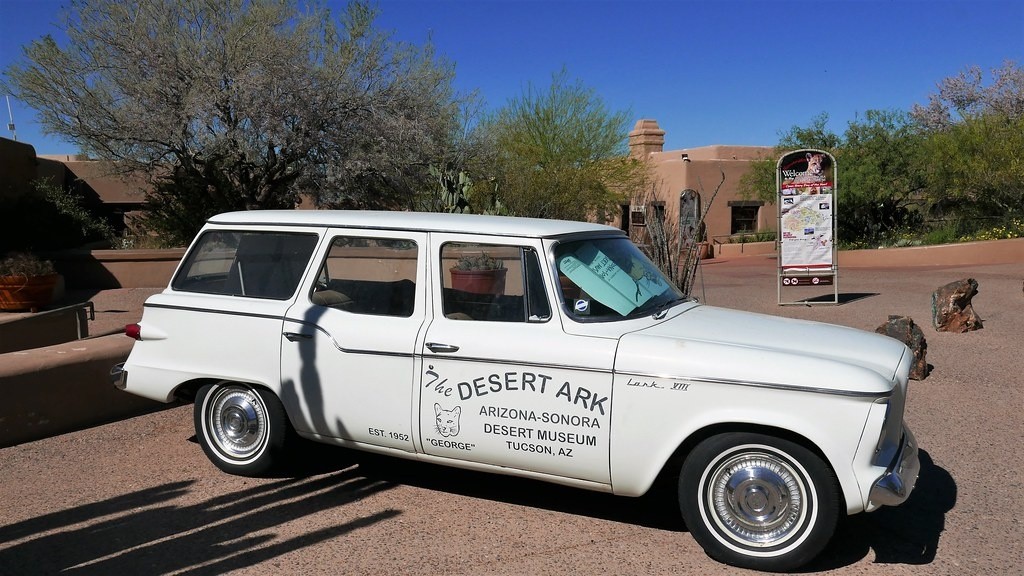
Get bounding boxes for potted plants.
[449,247,508,295]
[0,252,66,313]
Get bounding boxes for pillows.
[312,290,356,312]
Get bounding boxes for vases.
[694,241,709,260]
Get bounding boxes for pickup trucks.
[109,209,921,572]
